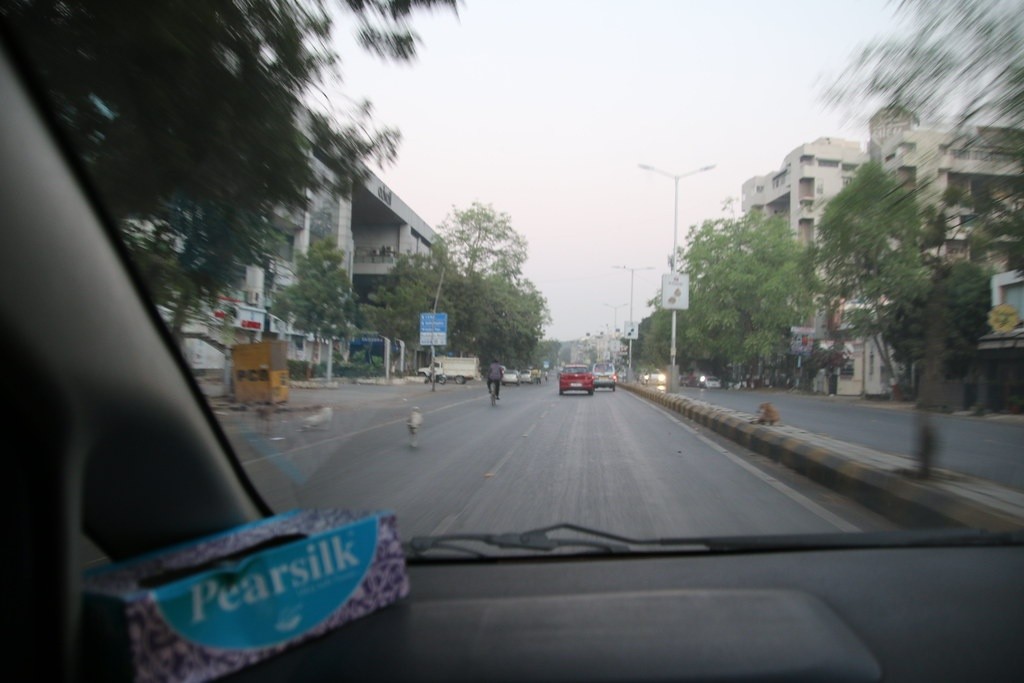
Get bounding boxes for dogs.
[750,401,782,425]
[299,406,334,429]
[406,407,423,447]
[254,400,289,436]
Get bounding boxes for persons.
[487,359,502,400]
[536,369,541,384]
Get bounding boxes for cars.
[502,370,520,386]
[559,365,595,396]
[700,377,721,390]
[640,368,666,385]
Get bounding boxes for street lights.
[603,303,627,363]
[613,266,656,383]
[637,160,720,394]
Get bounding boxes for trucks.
[419,355,480,385]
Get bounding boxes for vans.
[520,369,534,383]
[592,362,615,391]
[680,375,700,387]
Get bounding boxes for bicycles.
[489,382,499,407]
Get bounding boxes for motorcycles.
[424,371,446,384]
